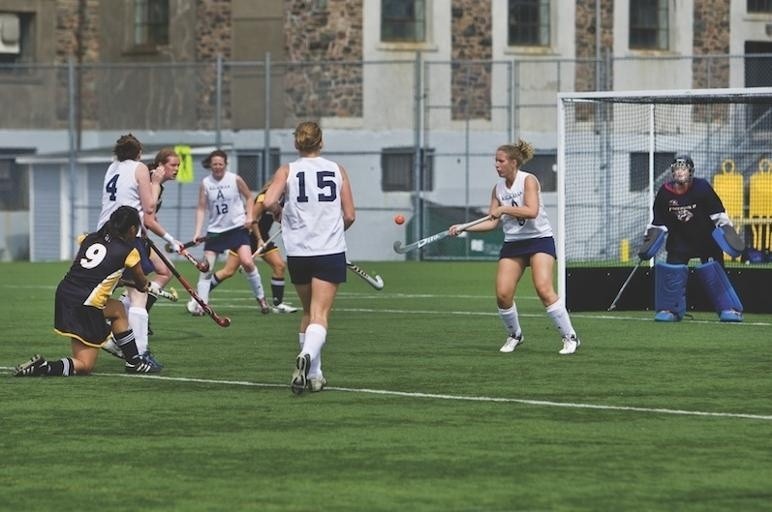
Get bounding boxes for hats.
[202,150,227,169]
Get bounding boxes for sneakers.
[500,332,525,353]
[192,304,207,317]
[261,302,300,315]
[558,335,581,355]
[101,336,165,375]
[12,353,48,377]
[289,351,327,396]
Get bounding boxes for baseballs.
[395,216,405,224]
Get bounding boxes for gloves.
[162,232,184,254]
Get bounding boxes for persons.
[14,206,162,378]
[448,138,582,355]
[193,151,272,318]
[106,148,186,335]
[637,153,745,321]
[95,131,166,358]
[210,171,300,314]
[262,120,358,395]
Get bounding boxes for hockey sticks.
[178,246,210,273]
[393,214,494,255]
[165,238,208,254]
[345,260,385,291]
[607,257,642,312]
[144,236,233,328]
[120,279,180,303]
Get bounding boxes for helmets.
[670,154,696,184]
